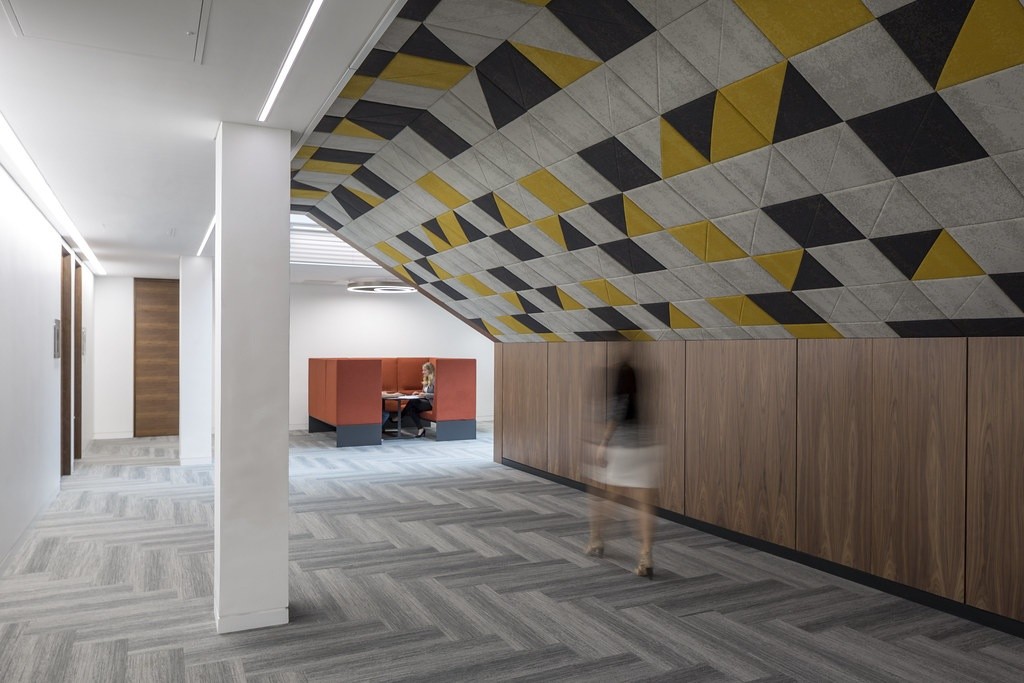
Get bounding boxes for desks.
[385,395,419,438]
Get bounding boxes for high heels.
[634,555,654,580]
[583,539,603,557]
[415,429,425,438]
[388,418,398,424]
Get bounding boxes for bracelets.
[603,436,610,442]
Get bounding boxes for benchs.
[402,404,436,421]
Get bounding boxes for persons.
[382,361,437,438]
[584,334,661,583]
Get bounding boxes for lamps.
[347,282,417,294]
